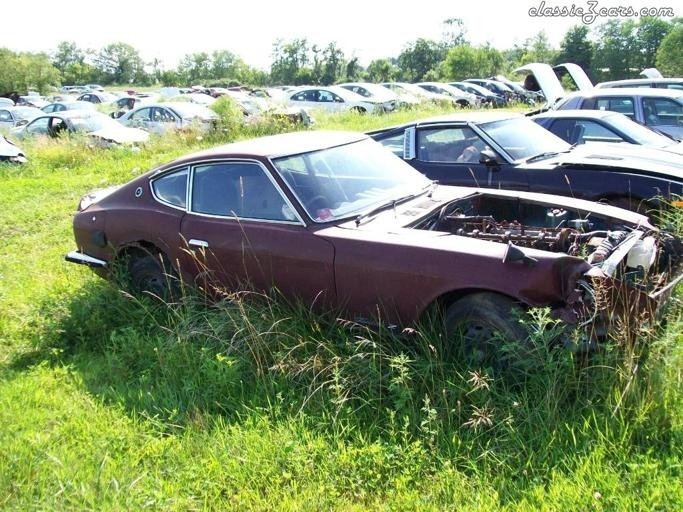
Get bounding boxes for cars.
[512,63,682,156]
[64,130,682,394]
[363,112,682,273]
[0,74,544,166]
